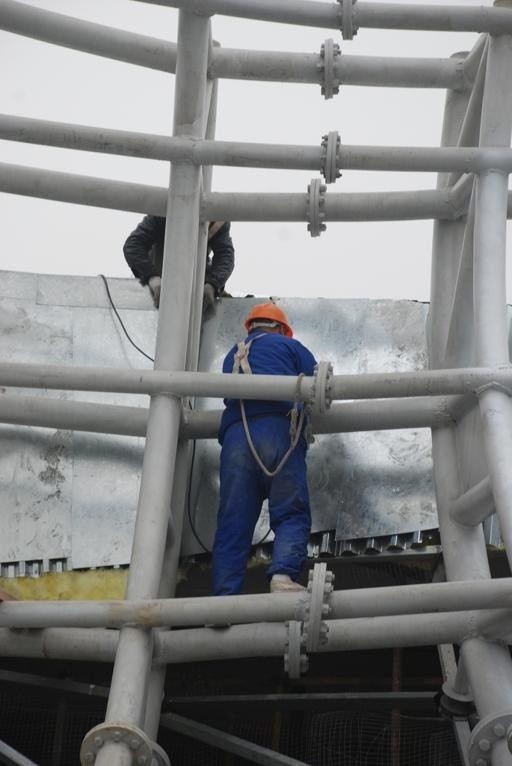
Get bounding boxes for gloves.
[148,276,161,306]
[201,283,215,314]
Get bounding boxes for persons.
[204,303,321,629]
[123,214,236,312]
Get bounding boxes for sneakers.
[268,575,307,593]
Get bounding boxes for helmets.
[242,302,293,338]
